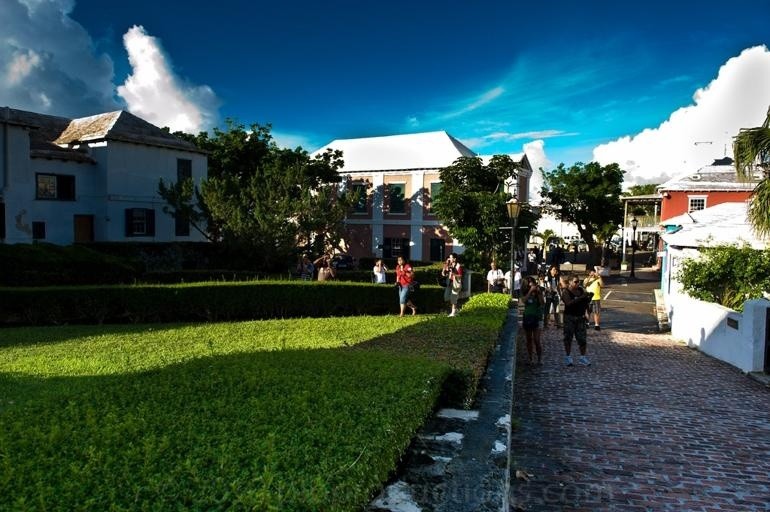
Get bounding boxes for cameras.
[535,284,545,295]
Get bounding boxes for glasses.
[573,281,578,283]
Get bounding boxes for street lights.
[630,217,639,281]
[503,196,528,298]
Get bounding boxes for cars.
[315,254,356,271]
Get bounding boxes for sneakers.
[579,355,592,366]
[565,355,574,365]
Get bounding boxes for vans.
[565,235,580,244]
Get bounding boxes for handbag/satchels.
[408,281,420,293]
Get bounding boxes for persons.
[372,260,389,285]
[296,253,313,280]
[313,255,334,281]
[487,258,605,367]
[445,256,450,264]
[392,258,417,315]
[441,252,463,318]
